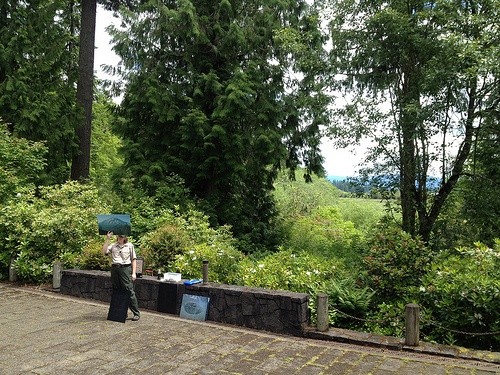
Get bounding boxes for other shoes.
[132,315,140,321]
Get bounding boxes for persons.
[101,231,141,321]
